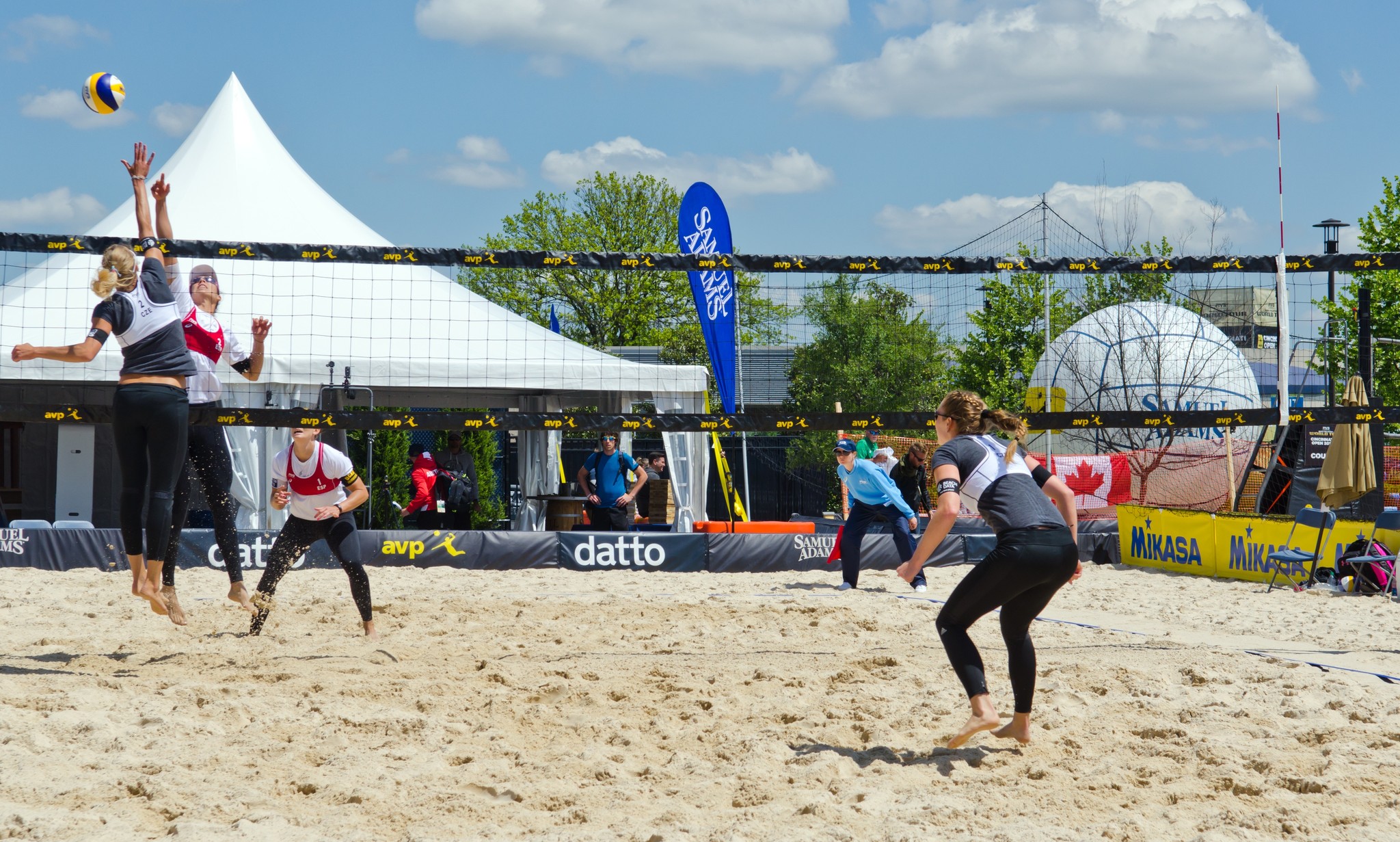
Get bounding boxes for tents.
[0,69,709,530]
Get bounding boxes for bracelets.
[131,174,148,181]
[333,504,342,514]
[254,337,265,343]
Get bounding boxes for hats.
[189,264,218,287]
[291,406,309,410]
[867,429,881,433]
[833,439,856,452]
[448,430,462,438]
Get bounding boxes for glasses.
[870,431,879,435]
[835,450,850,456]
[934,411,958,423]
[128,250,138,272]
[191,276,219,289]
[449,436,460,441]
[602,436,616,440]
[912,452,925,462]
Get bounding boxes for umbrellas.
[1315,375,1377,510]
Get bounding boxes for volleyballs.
[81,72,125,114]
[1337,576,1360,594]
[1023,301,1262,513]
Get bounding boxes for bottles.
[1391,580,1397,596]
[1327,572,1336,586]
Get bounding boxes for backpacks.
[1337,538,1396,594]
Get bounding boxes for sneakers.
[915,585,926,592]
[838,582,852,590]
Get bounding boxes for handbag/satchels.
[436,464,472,504]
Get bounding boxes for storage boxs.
[626,479,675,525]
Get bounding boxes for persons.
[586,446,597,494]
[895,389,1082,750]
[854,430,888,466]
[430,430,480,530]
[147,170,272,625]
[889,441,934,535]
[577,431,648,532]
[832,438,928,594]
[398,443,446,530]
[635,451,665,520]
[10,141,197,615]
[246,406,380,642]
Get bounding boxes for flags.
[1031,453,1133,511]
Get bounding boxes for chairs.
[1267,507,1336,591]
[9,520,52,528]
[1345,510,1400,597]
[52,521,95,529]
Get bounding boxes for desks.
[526,495,589,531]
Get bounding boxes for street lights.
[1314,218,1351,427]
